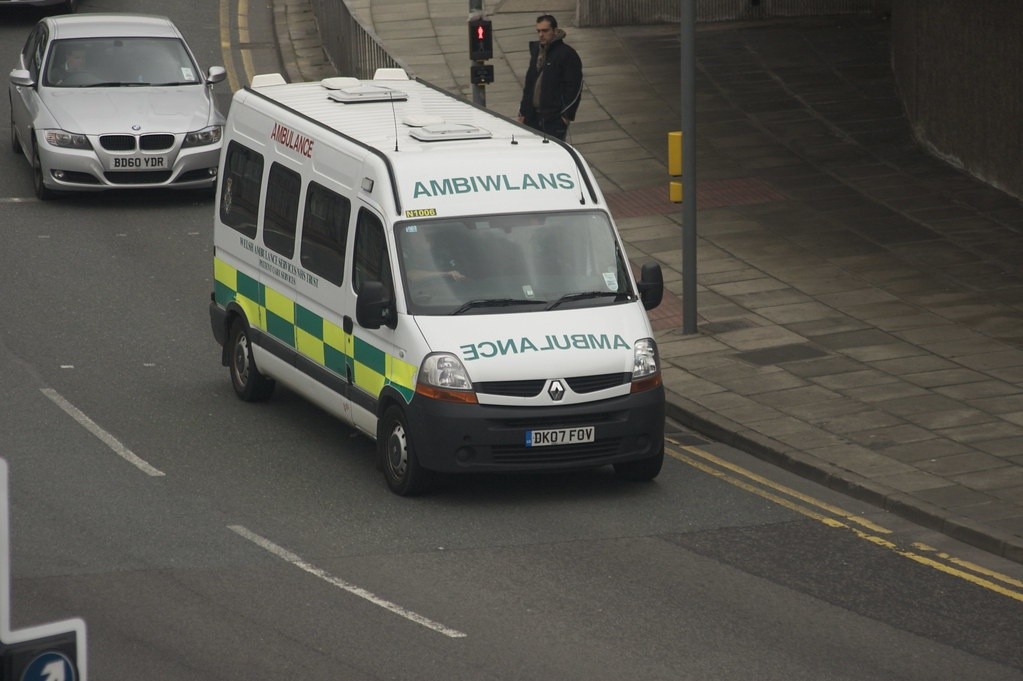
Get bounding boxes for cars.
[8,12,228,200]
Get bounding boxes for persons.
[517,15,583,141]
[401,228,465,284]
[51,45,96,84]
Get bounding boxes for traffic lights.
[467,17,493,59]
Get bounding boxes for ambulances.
[209,66,670,502]
